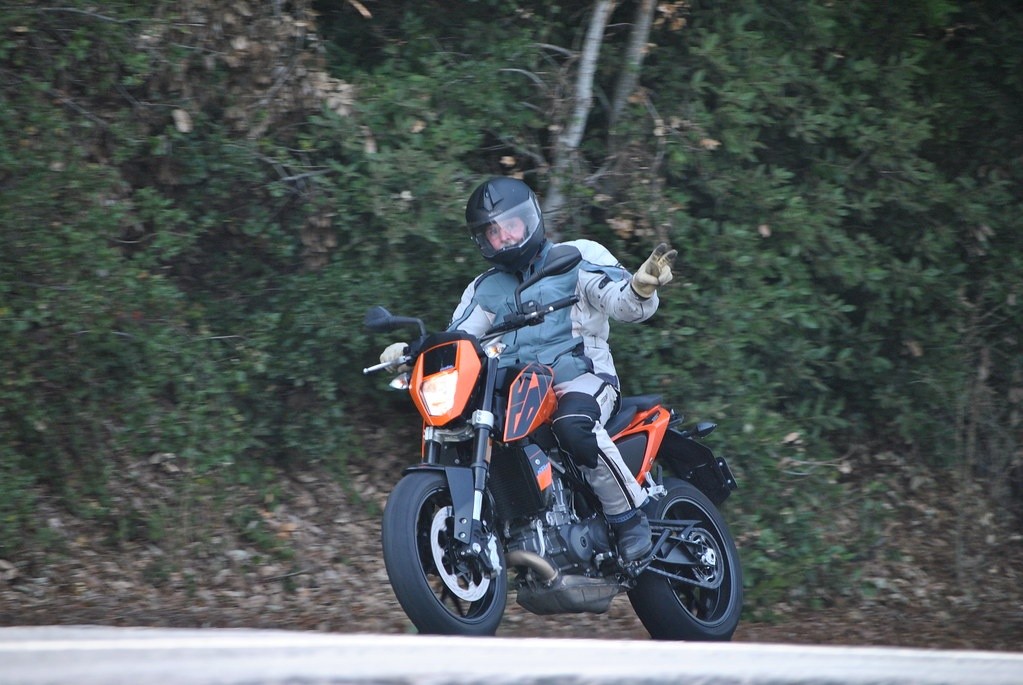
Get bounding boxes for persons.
[380,176,678,561]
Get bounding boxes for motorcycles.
[362,245,743,641]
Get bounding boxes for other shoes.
[614,511,653,561]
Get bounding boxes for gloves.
[631,243,680,300]
[380,342,411,373]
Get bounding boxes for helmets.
[466,176,547,274]
